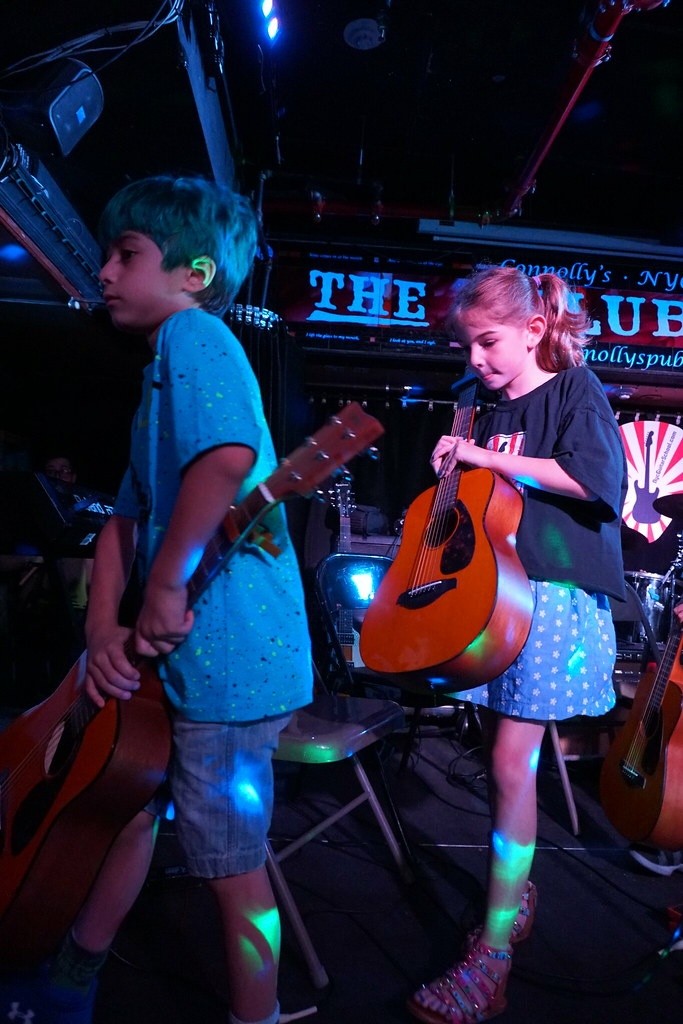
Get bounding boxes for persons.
[0,174,312,1024]
[10,455,86,604]
[406,268,683,1024]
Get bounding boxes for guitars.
[0,401,386,976]
[328,476,365,700]
[358,363,537,699]
[599,618,682,851]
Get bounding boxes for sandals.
[467,880,538,947]
[409,940,511,1024]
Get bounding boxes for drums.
[613,569,683,646]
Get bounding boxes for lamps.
[224,302,282,335]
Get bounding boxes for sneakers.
[272,982,316,1024]
[629,846,682,876]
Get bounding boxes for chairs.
[262,550,483,991]
[547,571,662,837]
